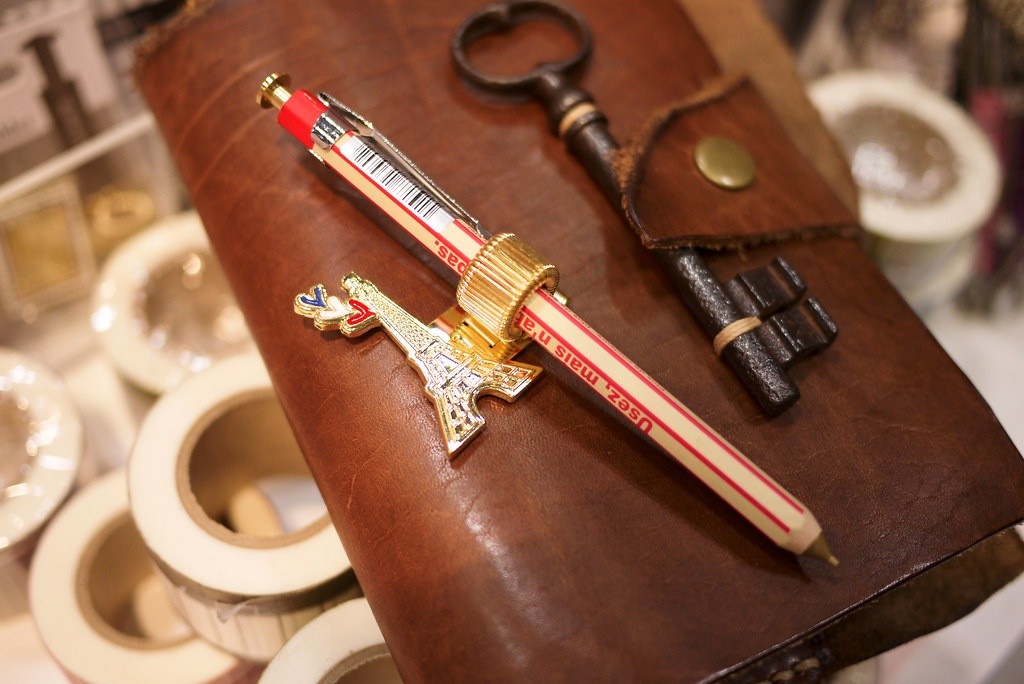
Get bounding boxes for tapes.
[807,66,1004,269]
[1,210,399,684]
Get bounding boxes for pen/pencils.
[256,72,840,571]
